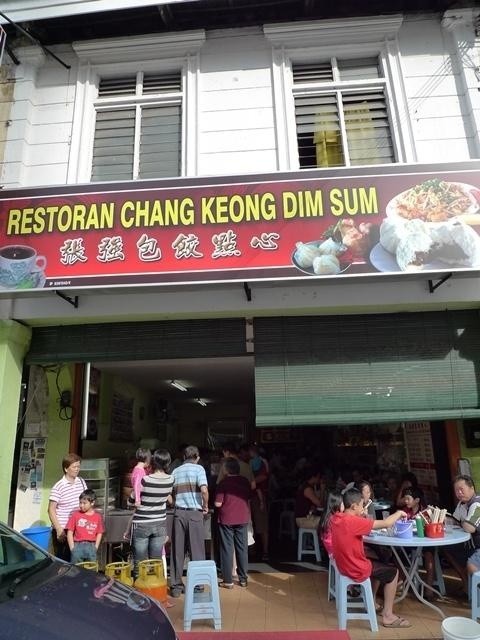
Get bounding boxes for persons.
[65,488,107,569]
[214,439,421,561]
[214,458,251,589]
[121,446,151,541]
[164,446,212,599]
[316,478,369,598]
[392,485,437,602]
[126,448,176,581]
[328,495,414,629]
[464,548,480,590]
[438,475,480,602]
[48,454,89,564]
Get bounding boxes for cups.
[386,526,395,536]
[0,243,50,287]
[382,511,390,521]
[424,524,444,538]
[366,513,374,520]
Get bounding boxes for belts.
[174,506,203,513]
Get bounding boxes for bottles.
[415,513,424,538]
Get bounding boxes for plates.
[1,264,48,290]
[384,180,480,227]
[289,239,353,275]
[368,227,479,274]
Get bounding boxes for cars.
[0,521,178,640]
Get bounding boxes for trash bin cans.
[20,527,51,552]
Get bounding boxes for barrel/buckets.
[20,526,53,562]
[395,518,414,539]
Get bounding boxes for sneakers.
[419,594,436,603]
[170,583,186,598]
[239,577,248,587]
[194,585,201,594]
[455,586,468,597]
[347,587,361,597]
[219,581,233,589]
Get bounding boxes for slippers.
[383,615,412,629]
[376,604,384,616]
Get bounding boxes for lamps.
[194,392,209,408]
[170,377,191,392]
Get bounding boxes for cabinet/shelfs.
[78,457,122,573]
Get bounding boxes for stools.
[183,560,222,633]
[280,510,297,541]
[337,572,380,632]
[297,528,322,562]
[411,547,447,595]
[327,558,366,611]
[471,571,480,622]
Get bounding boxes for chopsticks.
[417,508,448,524]
